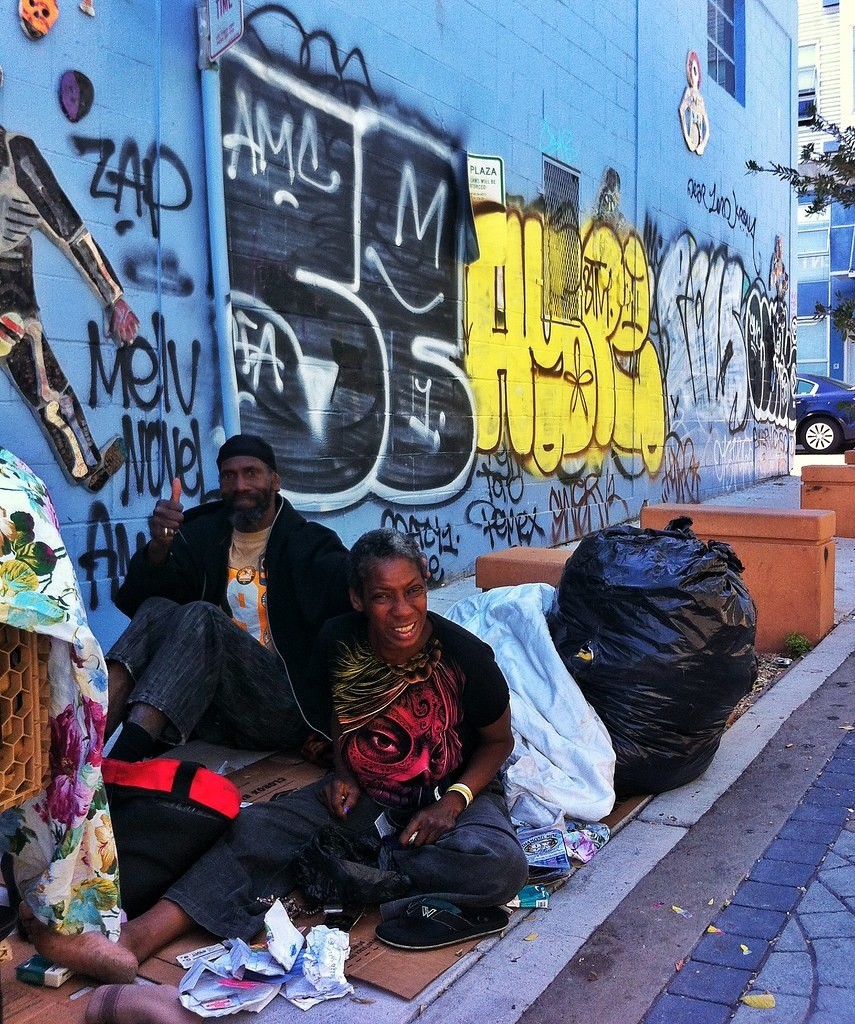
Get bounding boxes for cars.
[795,372,855,453]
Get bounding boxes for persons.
[21,528,528,982]
[101,434,349,761]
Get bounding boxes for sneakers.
[374,903,511,950]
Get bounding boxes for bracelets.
[446,782,473,810]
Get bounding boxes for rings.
[164,528,167,538]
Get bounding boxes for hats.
[216,434,277,470]
